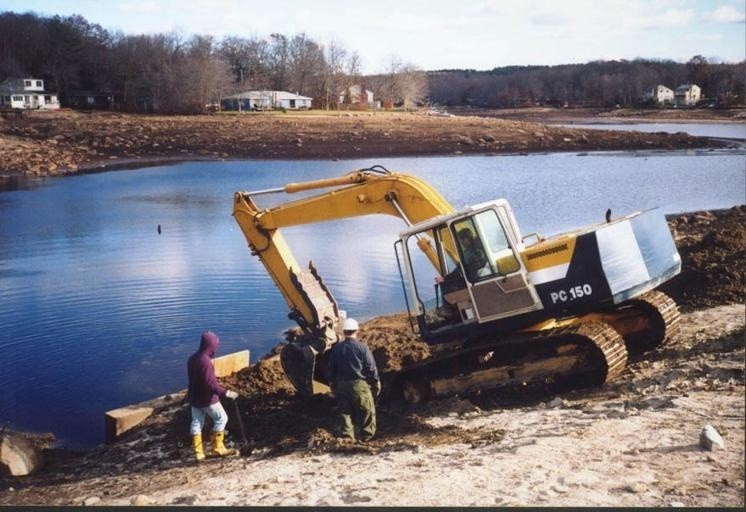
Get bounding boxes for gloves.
[330,383,337,393]
[226,391,239,401]
[374,384,381,396]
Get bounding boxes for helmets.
[342,319,359,331]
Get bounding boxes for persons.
[187,331,240,462]
[433,227,488,321]
[327,317,382,442]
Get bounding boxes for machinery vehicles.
[231,165,684,407]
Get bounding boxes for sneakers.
[437,303,457,314]
[363,432,372,442]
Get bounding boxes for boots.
[193,433,211,461]
[210,431,235,457]
